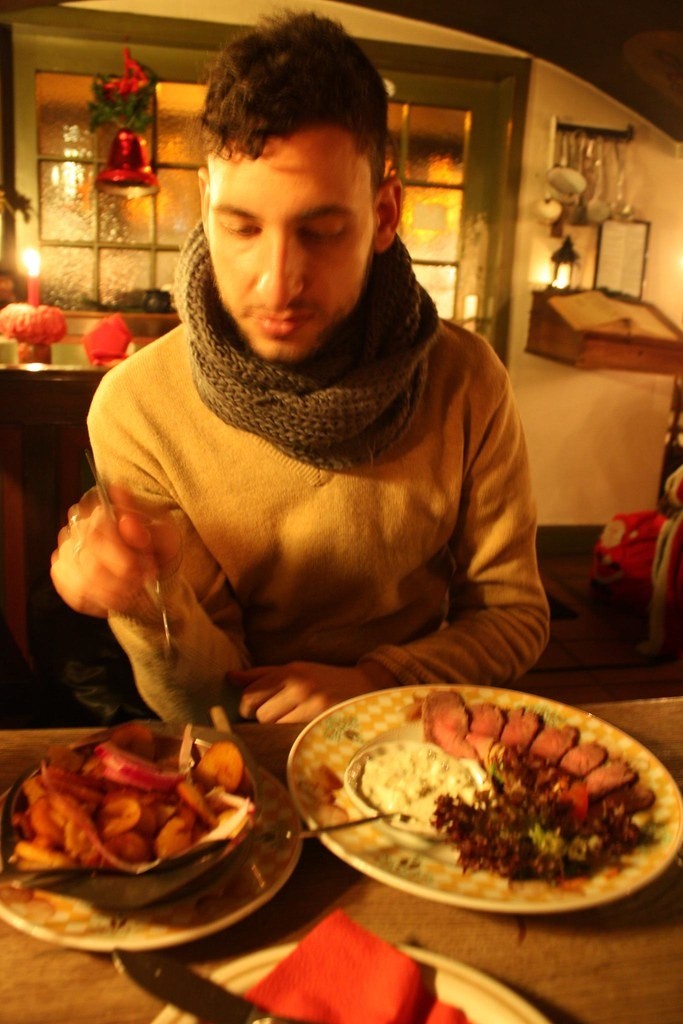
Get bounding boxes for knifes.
[112,947,301,1024]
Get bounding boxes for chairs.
[0,364,154,727]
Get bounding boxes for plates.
[286,683,683,915]
[0,757,304,954]
[154,942,550,1024]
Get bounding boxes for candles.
[28,272,40,305]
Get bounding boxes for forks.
[84,450,179,665]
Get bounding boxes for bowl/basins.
[6,734,258,912]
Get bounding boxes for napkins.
[243,910,475,1024]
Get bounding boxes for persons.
[50,10,551,726]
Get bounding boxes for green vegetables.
[429,745,659,893]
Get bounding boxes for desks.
[0,695,682,1024]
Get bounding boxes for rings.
[72,541,86,558]
